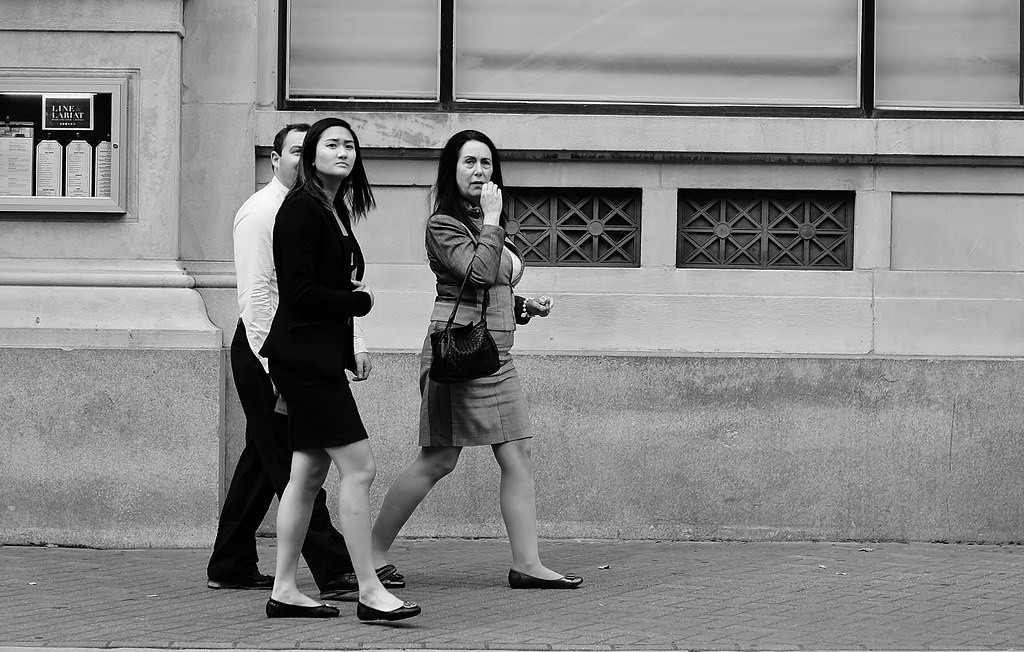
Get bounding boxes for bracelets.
[521,297,536,317]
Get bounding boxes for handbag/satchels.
[428,263,500,381]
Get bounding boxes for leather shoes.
[320,565,395,599]
[356,600,421,621]
[509,568,583,589]
[206,570,275,589]
[266,597,340,618]
[380,571,406,588]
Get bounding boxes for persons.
[371,130,584,589]
[205,117,421,621]
